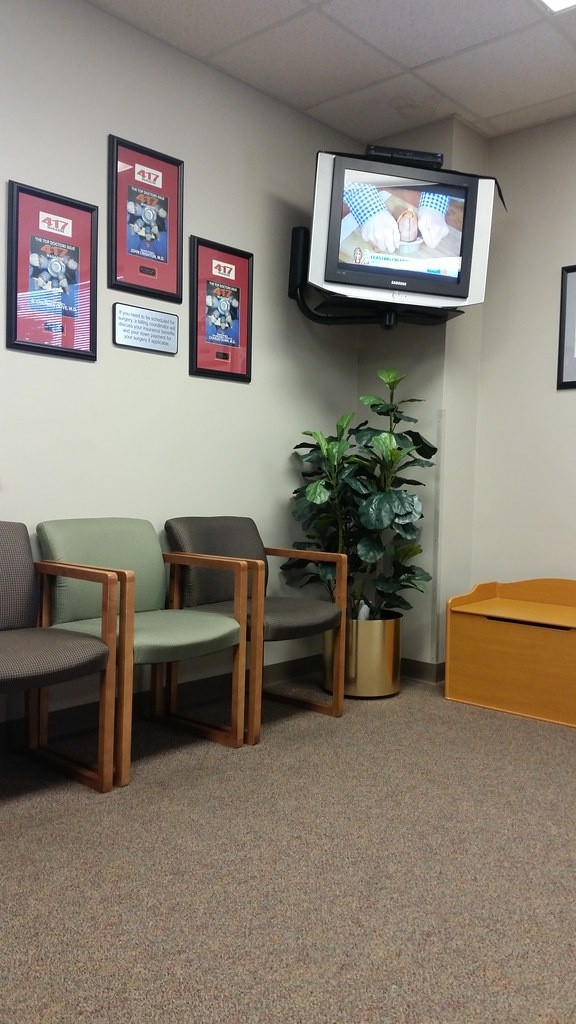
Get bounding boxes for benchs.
[442,578,575,728]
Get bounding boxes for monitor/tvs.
[308,151,495,309]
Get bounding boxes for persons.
[342,181,450,253]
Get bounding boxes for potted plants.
[281,367,437,696]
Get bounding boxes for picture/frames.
[557,265,576,389]
[189,235,254,383]
[6,179,99,360]
[108,134,184,304]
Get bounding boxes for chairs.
[37,517,247,786]
[0,520,119,793]
[165,516,347,744]
